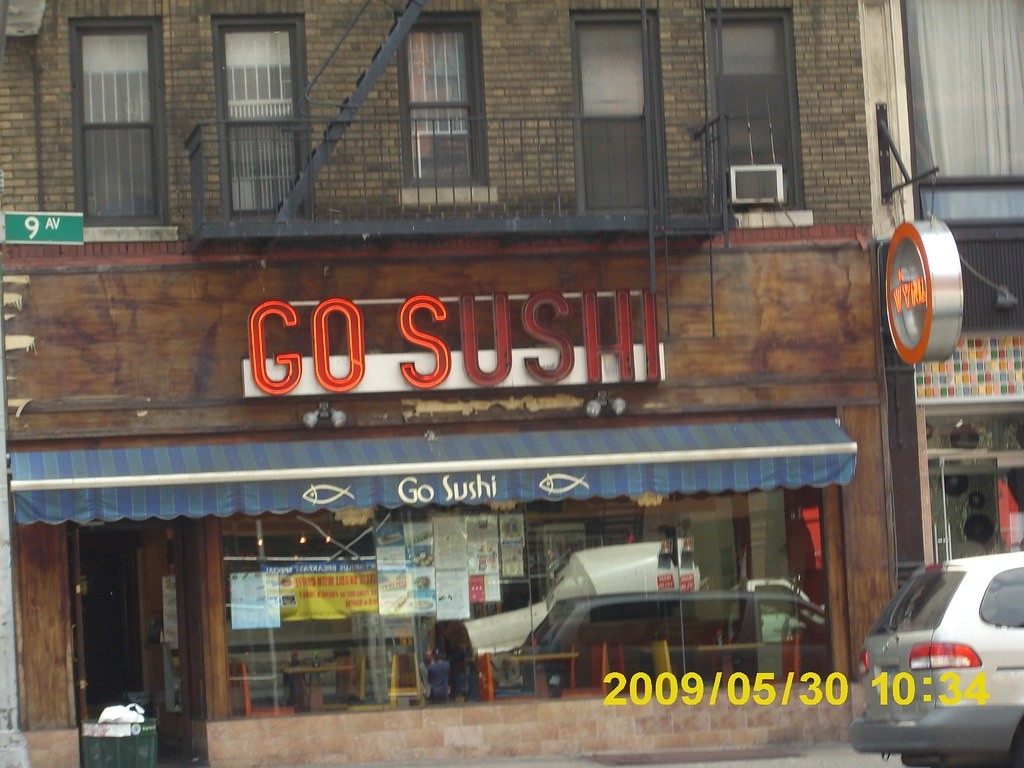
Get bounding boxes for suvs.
[847,551,1024,768]
[516,578,832,699]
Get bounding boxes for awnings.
[9,418,859,527]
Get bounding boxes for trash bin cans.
[80,716,159,768]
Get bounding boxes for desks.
[696,643,765,687]
[283,664,355,712]
[506,651,582,700]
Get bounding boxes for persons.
[419,648,451,703]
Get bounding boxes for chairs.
[323,653,425,714]
[473,634,803,703]
[237,659,297,719]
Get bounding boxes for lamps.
[583,390,627,419]
[301,402,347,431]
[958,251,1019,311]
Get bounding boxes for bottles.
[312,648,320,668]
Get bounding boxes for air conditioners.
[729,164,784,204]
